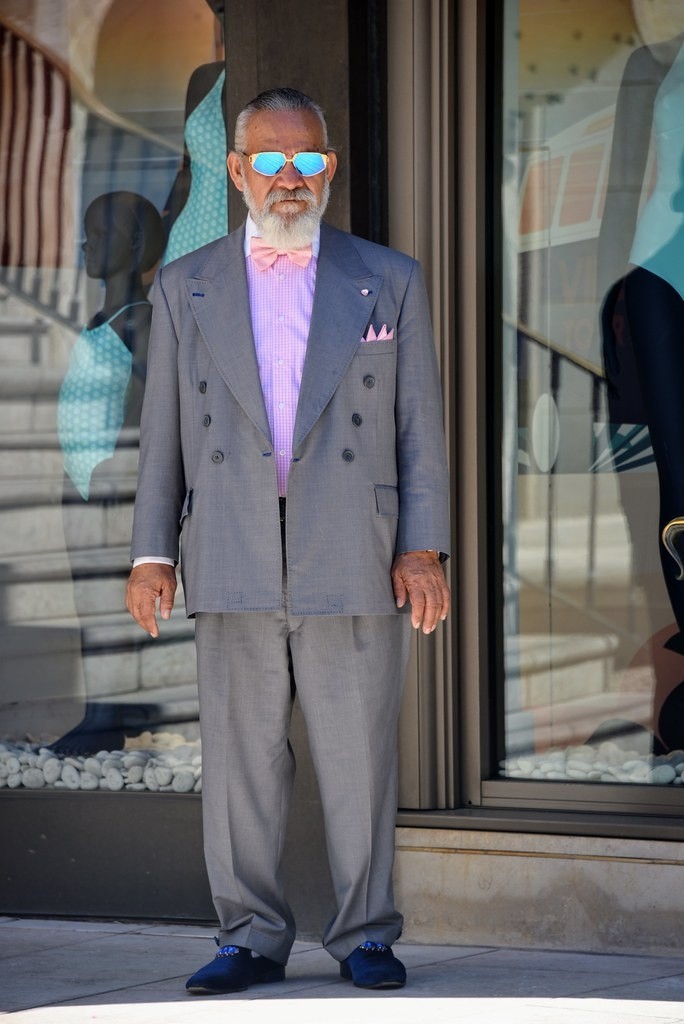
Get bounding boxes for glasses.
[242,152,329,178]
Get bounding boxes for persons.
[125,88,453,996]
[34,191,168,759]
[593,36,684,673]
[158,0,228,265]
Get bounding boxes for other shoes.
[185,945,285,994]
[339,941,407,988]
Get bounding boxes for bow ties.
[250,236,312,271]
